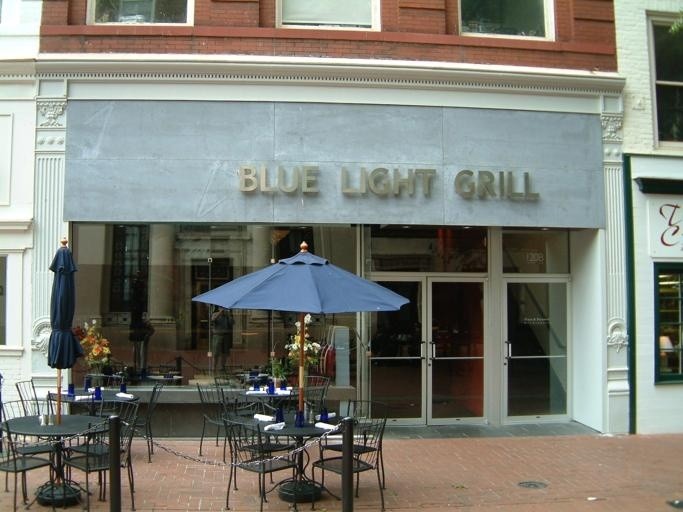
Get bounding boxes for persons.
[209,306,233,375]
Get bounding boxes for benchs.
[290,326,351,385]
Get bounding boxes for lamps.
[660,336,675,366]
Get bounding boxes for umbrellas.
[47,239,83,425]
[192,240,411,427]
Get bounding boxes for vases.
[303,363,308,385]
[94,365,102,386]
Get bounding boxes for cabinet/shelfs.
[660,286,679,327]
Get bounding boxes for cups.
[281,380,286,390]
[296,410,304,427]
[94,386,103,401]
[253,380,261,391]
[276,408,283,423]
[84,375,93,392]
[67,383,75,397]
[321,407,327,423]
[267,382,275,394]
[120,382,127,394]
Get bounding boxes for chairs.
[195,375,391,511]
[0,378,164,512]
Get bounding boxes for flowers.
[284,312,323,367]
[79,320,112,366]
